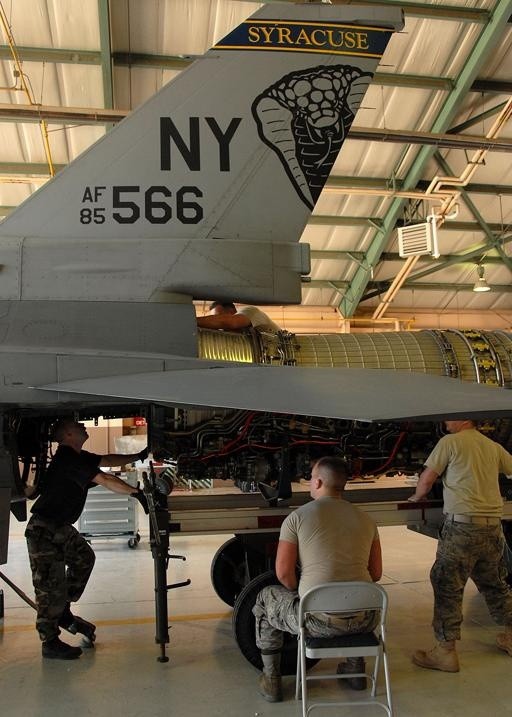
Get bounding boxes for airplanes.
[0,0,512,677]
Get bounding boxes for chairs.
[292,581,393,717]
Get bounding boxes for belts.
[311,609,372,626]
[445,512,500,525]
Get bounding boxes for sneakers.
[41,636,83,660]
[59,601,75,629]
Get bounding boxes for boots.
[412,638,460,674]
[335,656,367,691]
[494,624,512,657]
[256,651,285,704]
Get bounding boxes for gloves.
[130,486,151,515]
[138,443,152,463]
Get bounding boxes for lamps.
[471,258,492,293]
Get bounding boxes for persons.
[251,455,382,703]
[25,419,150,660]
[408,419,512,673]
